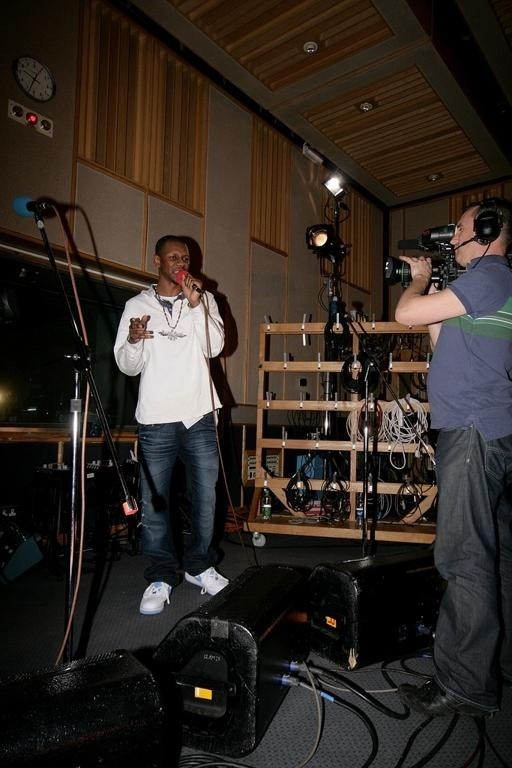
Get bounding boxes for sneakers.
[139,580,172,615]
[184,567,230,596]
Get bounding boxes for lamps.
[307,169,350,263]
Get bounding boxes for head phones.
[473,197,504,243]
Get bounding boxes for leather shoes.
[397,675,500,721]
[412,613,438,637]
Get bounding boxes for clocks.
[13,56,56,102]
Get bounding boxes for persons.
[113,233,232,616]
[393,197,512,718]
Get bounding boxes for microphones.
[453,239,474,252]
[13,196,48,216]
[175,271,204,294]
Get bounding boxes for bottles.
[261,490,272,521]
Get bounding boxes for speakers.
[152,565,314,758]
[0,649,180,766]
[311,551,448,668]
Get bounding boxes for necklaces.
[153,284,184,342]
